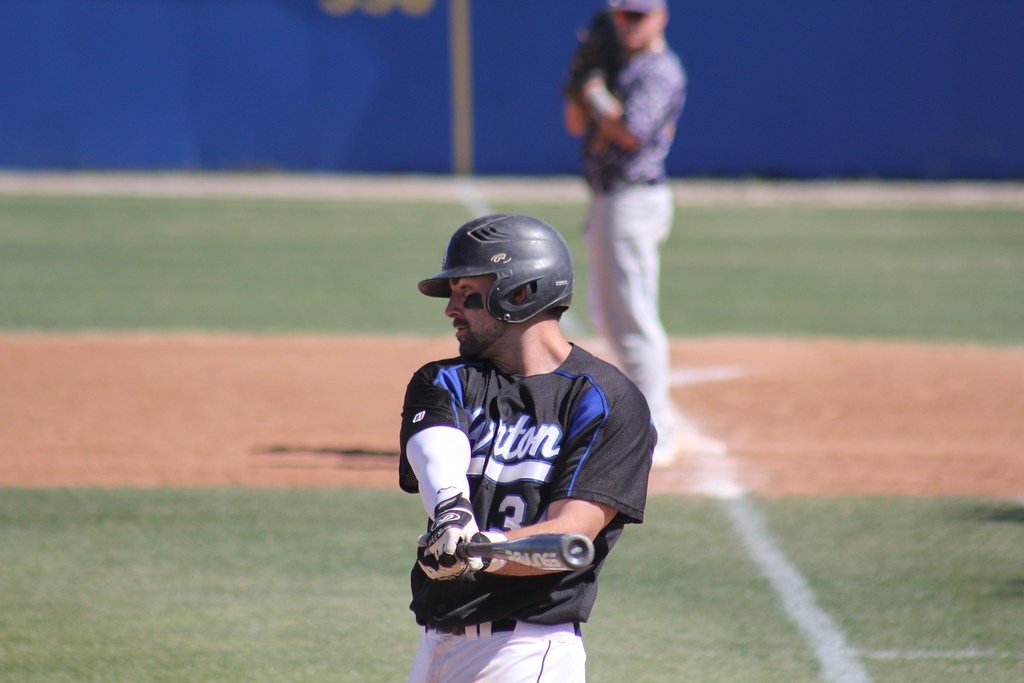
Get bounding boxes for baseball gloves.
[580,124,629,194]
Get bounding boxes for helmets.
[418,214,573,324]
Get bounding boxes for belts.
[425,619,582,636]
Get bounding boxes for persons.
[399,214,657,683]
[560,0,688,470]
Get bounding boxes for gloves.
[417,488,493,582]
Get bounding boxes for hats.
[607,0,668,13]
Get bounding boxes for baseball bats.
[456,533,596,572]
